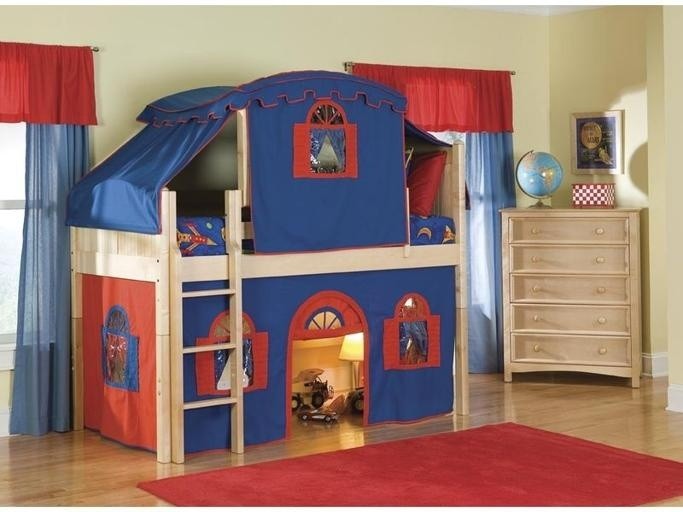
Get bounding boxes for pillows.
[405,148,447,216]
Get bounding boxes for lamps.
[338,331,365,390]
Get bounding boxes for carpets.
[137,421,683,507]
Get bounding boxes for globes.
[515,150,563,208]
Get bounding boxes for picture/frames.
[569,108,625,175]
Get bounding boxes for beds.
[68,70,469,463]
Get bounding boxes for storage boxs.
[571,183,616,209]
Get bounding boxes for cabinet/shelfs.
[498,206,643,388]
